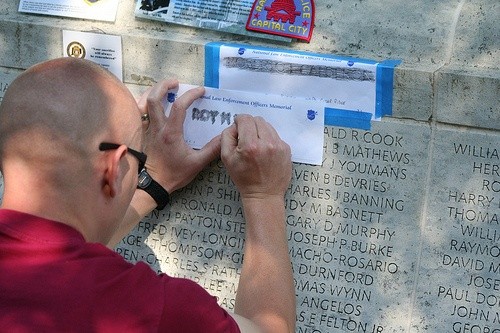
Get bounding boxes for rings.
[140,113,148,121]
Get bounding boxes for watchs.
[137,169,169,210]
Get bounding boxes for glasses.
[99,142,147,169]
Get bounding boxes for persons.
[0,58,294,333]
